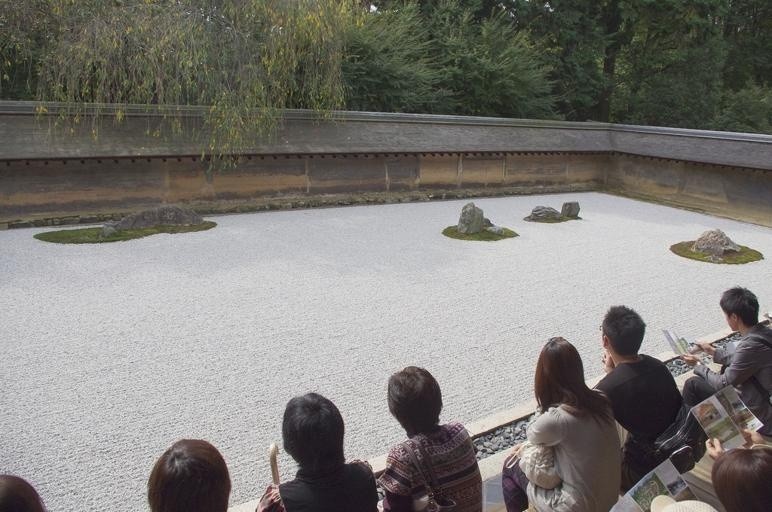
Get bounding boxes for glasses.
[598,324,609,338]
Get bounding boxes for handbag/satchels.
[518,442,563,488]
[377,497,457,512]
[652,404,710,474]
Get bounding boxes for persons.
[0,474,46,512]
[146,438,231,512]
[590,304,687,492]
[377,365,483,512]
[254,392,380,512]
[501,336,623,512]
[680,285,772,436]
[703,428,772,512]
